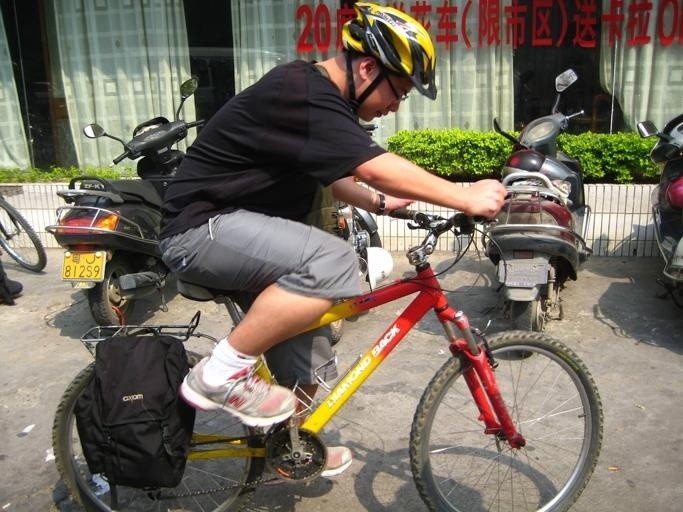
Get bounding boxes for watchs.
[374,194,386,217]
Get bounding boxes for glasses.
[383,74,409,101]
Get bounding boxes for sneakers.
[178,356,299,427]
[323,445,352,477]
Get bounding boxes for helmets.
[365,246,393,291]
[341,2,437,99]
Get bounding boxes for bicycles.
[56,209,604,509]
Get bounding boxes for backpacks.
[74,328,196,492]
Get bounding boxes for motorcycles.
[480,69,596,358]
[325,126,394,344]
[636,114,679,326]
[46,77,204,329]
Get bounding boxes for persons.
[158,0,510,479]
[0,249,25,306]
